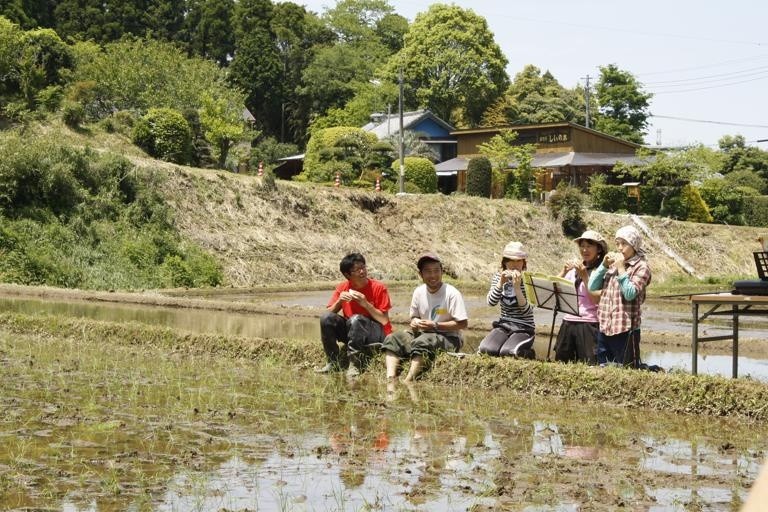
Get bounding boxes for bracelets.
[434,322,438,330]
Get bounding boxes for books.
[521,270,578,314]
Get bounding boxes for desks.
[688,292,768,379]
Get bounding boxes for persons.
[390,382,457,502]
[554,228,607,365]
[314,253,391,378]
[589,225,667,373]
[381,253,468,382]
[329,380,386,487]
[478,240,536,360]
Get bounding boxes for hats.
[573,230,608,253]
[417,252,440,269]
[503,241,528,260]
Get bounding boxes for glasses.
[348,264,365,273]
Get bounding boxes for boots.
[313,356,361,378]
